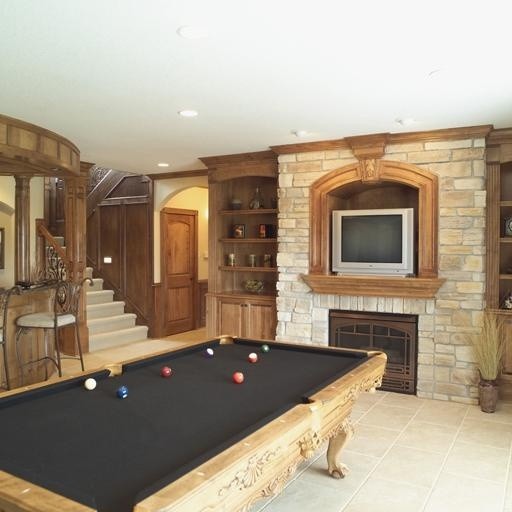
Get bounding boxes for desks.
[0,336,392,511]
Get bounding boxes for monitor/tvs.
[332,208,414,277]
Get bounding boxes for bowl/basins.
[230,202,242,211]
[243,281,265,294]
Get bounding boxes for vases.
[479,379,498,413]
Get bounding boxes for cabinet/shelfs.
[208,156,277,339]
[485,139,512,380]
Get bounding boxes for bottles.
[263,255,272,265]
[249,254,257,267]
[248,185,264,210]
[227,253,236,265]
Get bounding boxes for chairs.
[0,276,94,391]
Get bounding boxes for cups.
[233,223,246,238]
[260,223,277,239]
